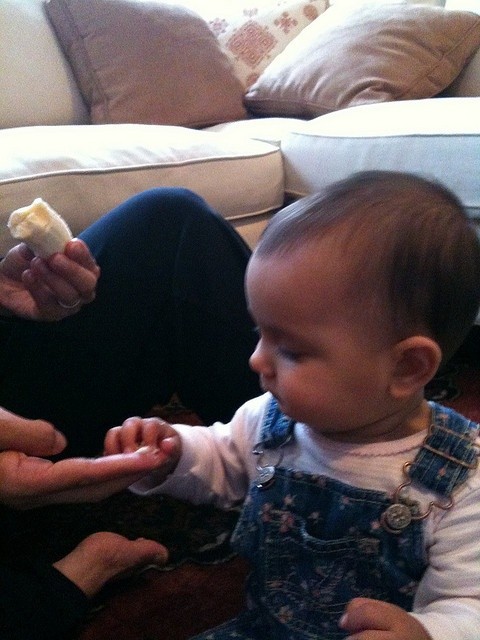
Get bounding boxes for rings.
[58,297,83,308]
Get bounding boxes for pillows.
[44,0,255,128]
[244,0,479,118]
[207,0,328,87]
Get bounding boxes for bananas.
[7,197,72,261]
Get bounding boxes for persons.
[105,168,480,640]
[0,184,267,640]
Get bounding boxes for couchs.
[1,0,480,250]
